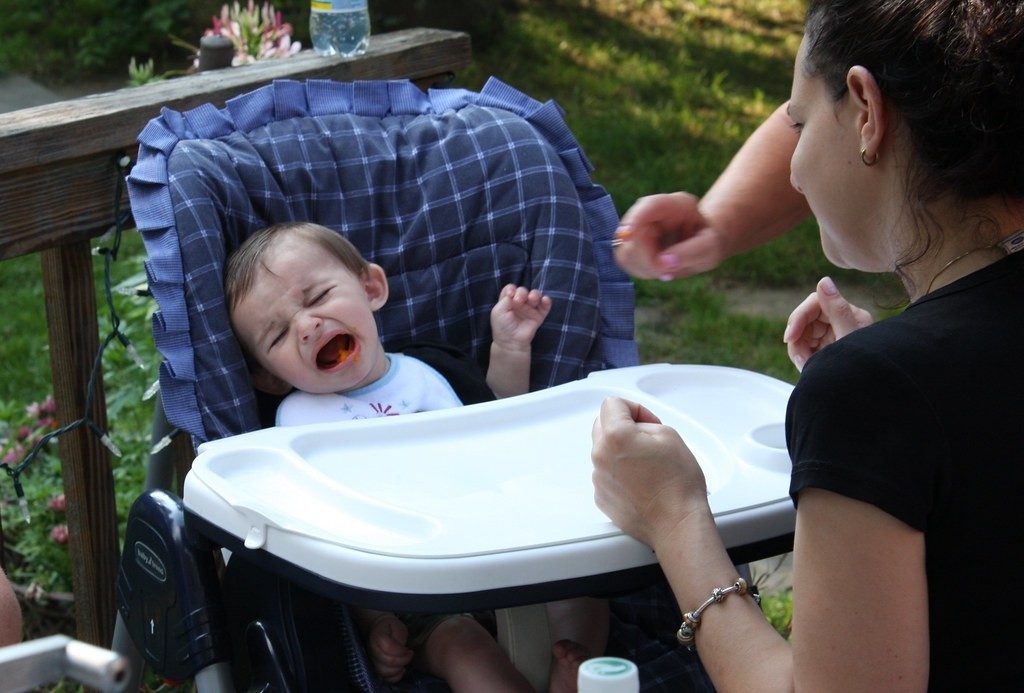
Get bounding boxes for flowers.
[202,0,301,66]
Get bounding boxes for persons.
[592,0,1024,693]
[612,99,812,281]
[225,222,608,693]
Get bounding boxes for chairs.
[123,76,798,693]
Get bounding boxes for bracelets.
[678,577,761,641]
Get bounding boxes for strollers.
[100,76,795,693]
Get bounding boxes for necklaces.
[925,245,997,294]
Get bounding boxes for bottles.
[307,0,370,59]
[575,656,640,693]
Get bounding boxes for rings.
[611,238,623,246]
